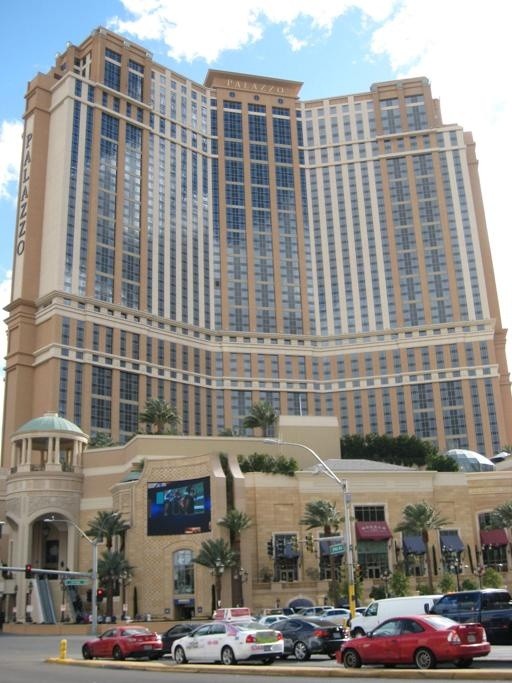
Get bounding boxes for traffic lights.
[25,564,31,577]
[267,539,272,554]
[277,537,283,552]
[289,535,299,551]
[96,588,102,601]
[338,563,347,584]
[355,563,363,582]
[305,535,312,550]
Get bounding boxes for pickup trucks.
[424,588,512,646]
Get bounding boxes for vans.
[349,594,444,639]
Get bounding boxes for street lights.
[210,557,225,609]
[43,512,121,634]
[56,566,74,619]
[116,570,133,619]
[379,543,504,603]
[264,438,357,623]
[232,567,248,607]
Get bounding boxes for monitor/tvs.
[147,476,212,536]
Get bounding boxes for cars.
[81,625,165,662]
[269,616,346,662]
[334,615,491,669]
[212,605,369,626]
[162,623,201,656]
[171,620,285,667]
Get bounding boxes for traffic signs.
[86,588,91,600]
[328,543,345,553]
[64,578,88,585]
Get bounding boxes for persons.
[76,613,117,624]
[163,485,197,505]
